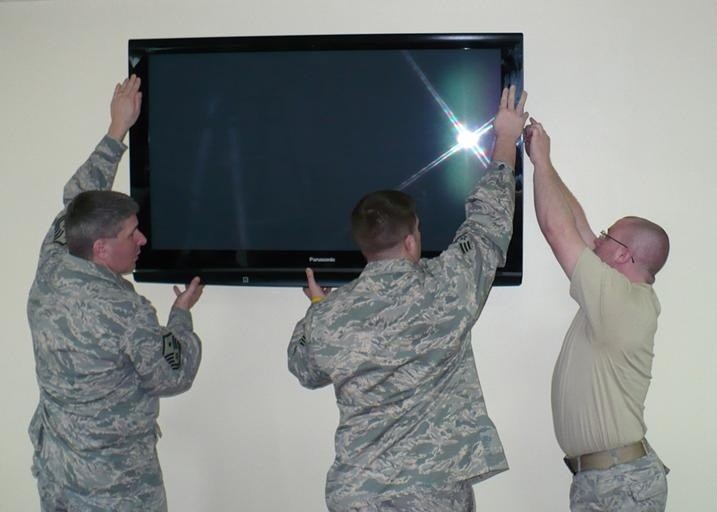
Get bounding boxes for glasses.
[599,231,634,263]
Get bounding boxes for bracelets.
[310,296,327,303]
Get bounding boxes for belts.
[564,437,651,476]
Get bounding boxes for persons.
[25,72,207,512]
[284,81,531,512]
[522,115,671,512]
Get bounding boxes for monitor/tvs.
[128,32,523,287]
